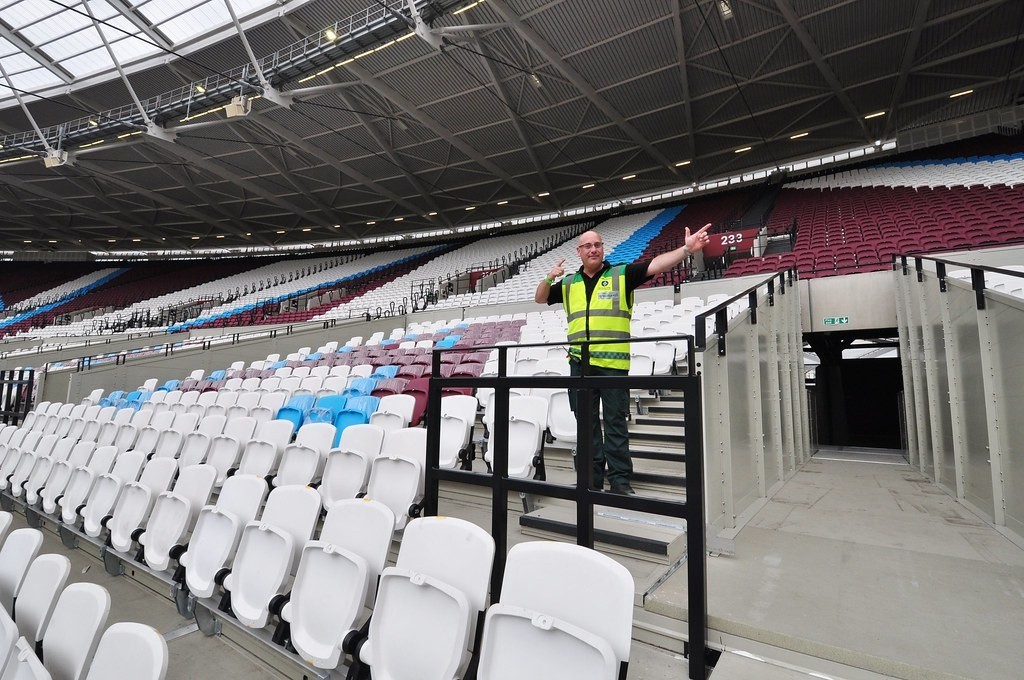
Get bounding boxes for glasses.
[578,242,604,249]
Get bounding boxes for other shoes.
[610,485,635,495]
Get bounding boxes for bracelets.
[544,273,555,284]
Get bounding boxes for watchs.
[682,245,694,256]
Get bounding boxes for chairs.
[0,150,1024,680]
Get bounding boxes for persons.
[535,223,713,497]
